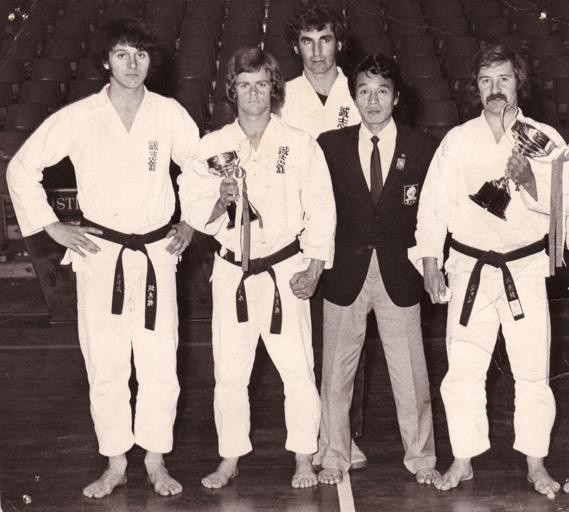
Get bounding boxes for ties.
[370,136,383,204]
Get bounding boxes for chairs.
[0,0,568,208]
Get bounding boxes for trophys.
[204,150,262,230]
[467,103,561,221]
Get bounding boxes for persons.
[419,44,568,495]
[279,7,369,473]
[177,47,339,488]
[6,17,200,498]
[316,55,442,485]
